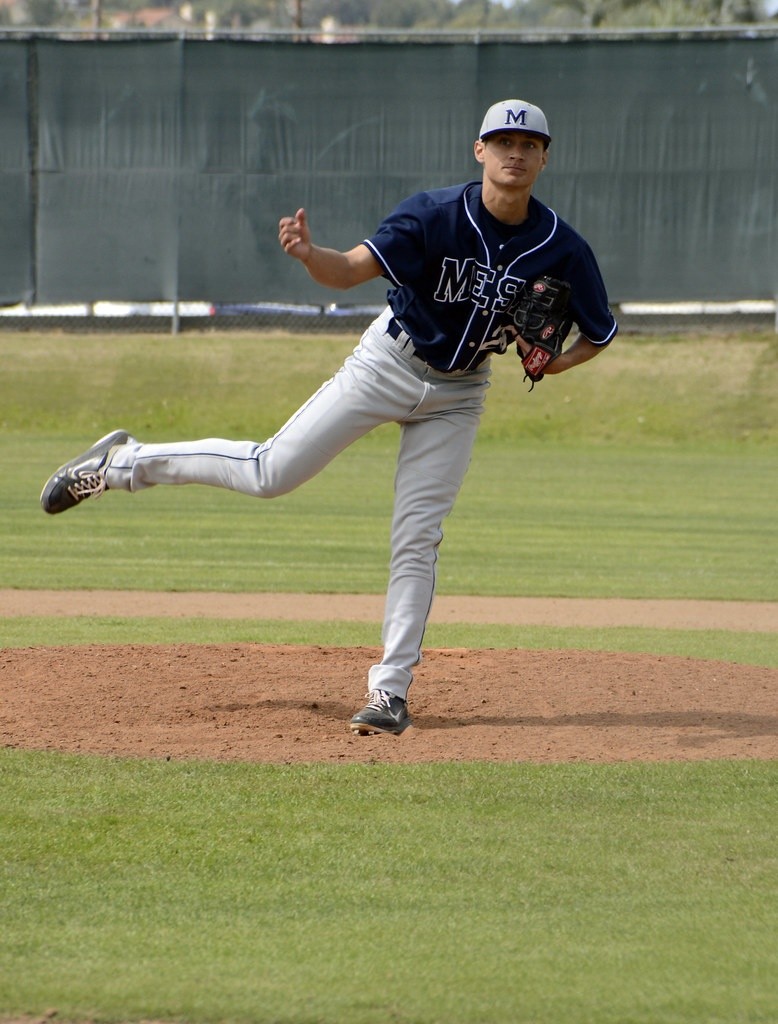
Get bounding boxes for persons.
[35,95,620,741]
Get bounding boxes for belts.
[388,320,455,374]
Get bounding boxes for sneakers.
[350,690,412,734]
[40,429,133,515]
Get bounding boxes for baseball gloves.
[512,274,573,382]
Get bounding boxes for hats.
[478,100,552,150]
